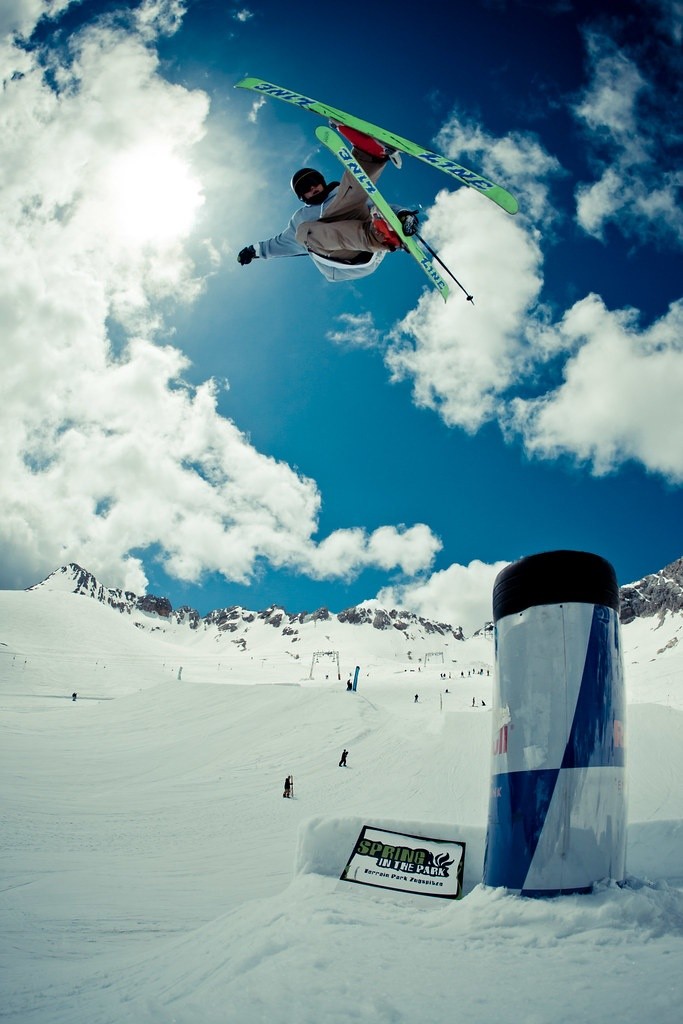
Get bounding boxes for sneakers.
[337,126,394,166]
[368,218,403,251]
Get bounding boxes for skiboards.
[234,77,520,304]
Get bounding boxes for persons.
[482,700,485,705]
[73,693,77,700]
[238,117,420,282]
[441,668,489,679]
[473,696,475,706]
[415,694,418,702]
[347,679,352,691]
[339,749,348,767]
[283,776,293,798]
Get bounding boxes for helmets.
[290,168,325,203]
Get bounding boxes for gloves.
[237,245,259,265]
[397,208,419,236]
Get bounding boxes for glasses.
[300,178,321,196]
